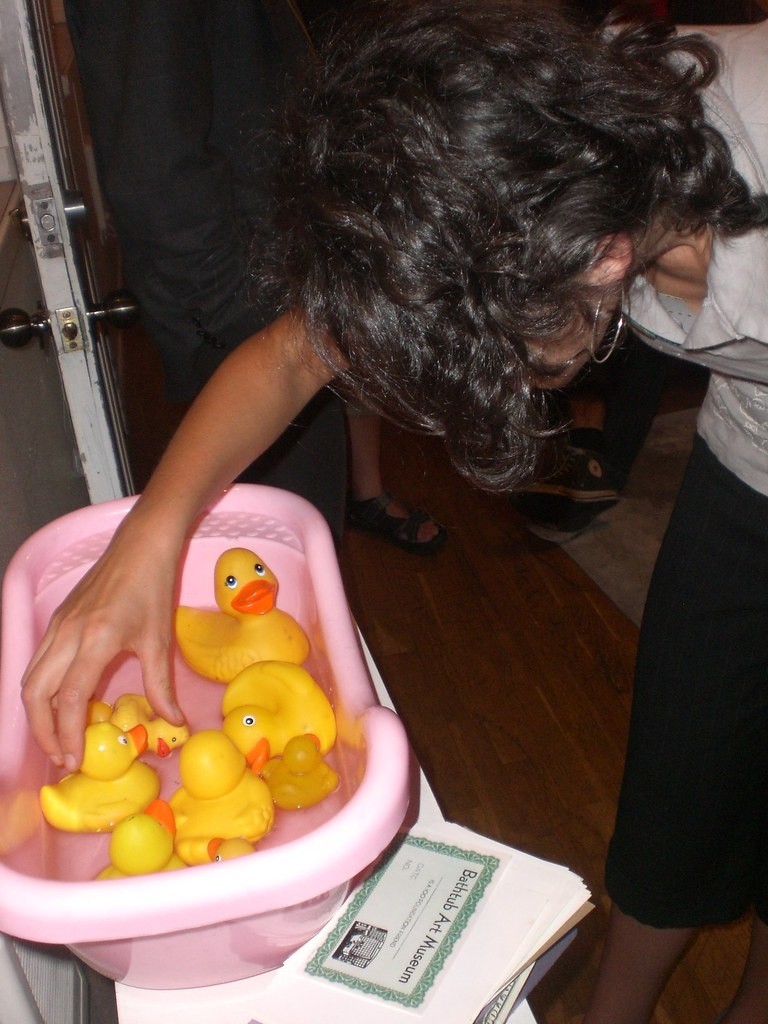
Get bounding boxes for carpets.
[559,407,700,629]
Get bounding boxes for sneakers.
[510,432,619,542]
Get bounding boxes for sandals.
[349,491,449,556]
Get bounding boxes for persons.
[63,0,345,558]
[507,333,668,542]
[346,393,448,553]
[21,15,768,1024]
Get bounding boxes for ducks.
[40,547,339,878]
